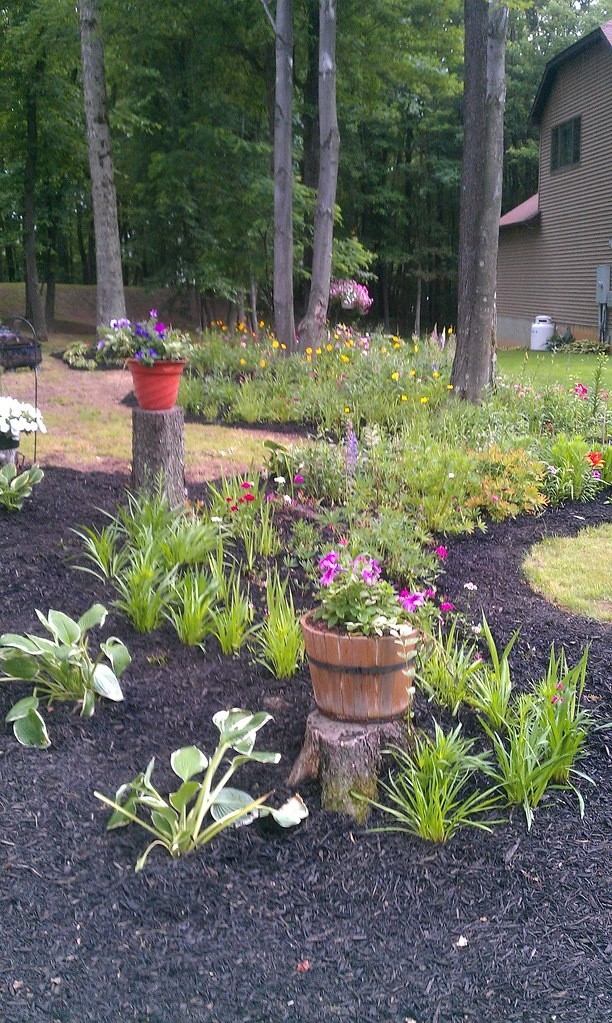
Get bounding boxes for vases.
[299,607,419,724]
[122,357,188,411]
[0,432,19,450]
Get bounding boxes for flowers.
[312,551,425,639]
[0,395,47,441]
[95,309,195,369]
